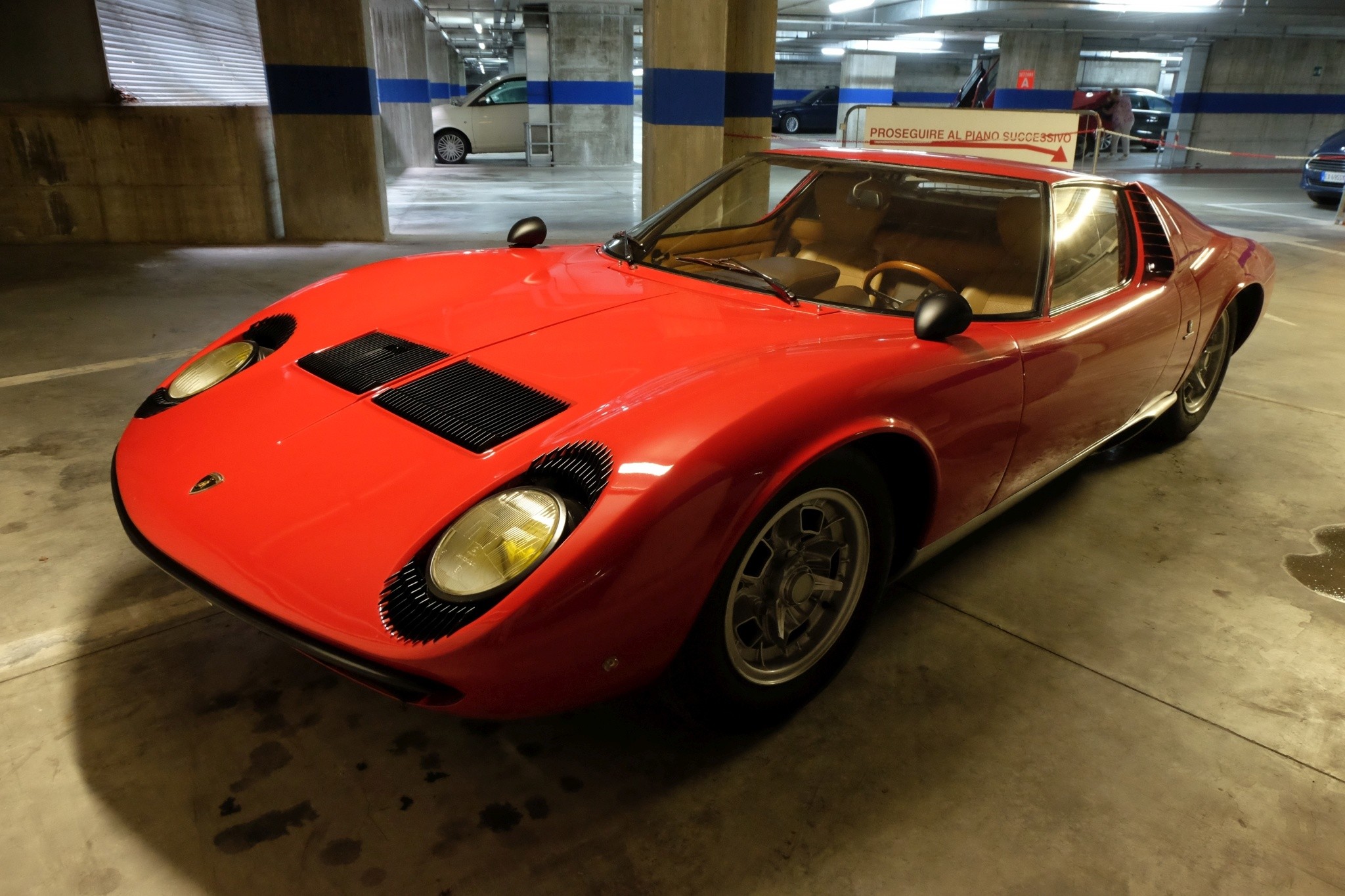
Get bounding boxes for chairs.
[796,175,892,304]
[960,196,1043,313]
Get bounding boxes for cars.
[1094,92,1174,152]
[431,72,549,164]
[772,84,897,134]
[1298,127,1345,207]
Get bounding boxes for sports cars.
[109,148,1275,725]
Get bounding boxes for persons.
[1102,88,1135,161]
